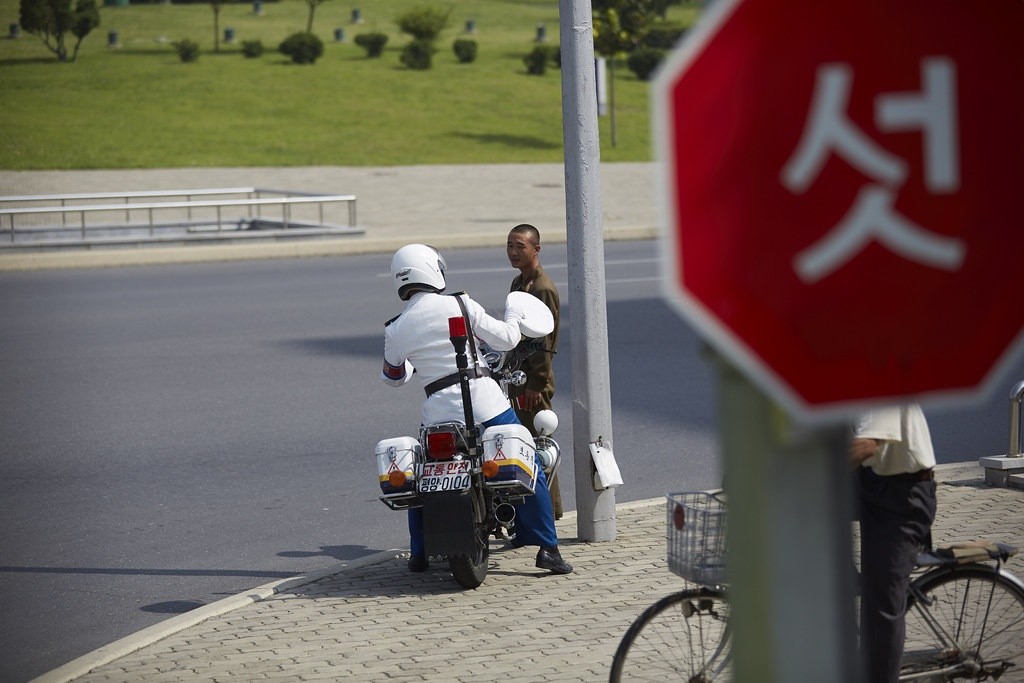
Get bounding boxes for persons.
[380,244,574,575]
[853,401,938,682]
[504,224,564,520]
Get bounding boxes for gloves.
[503,304,526,326]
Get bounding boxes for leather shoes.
[407,554,429,571]
[536,549,575,574]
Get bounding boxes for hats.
[505,291,555,342]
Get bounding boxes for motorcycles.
[374,337,566,592]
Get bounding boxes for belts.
[424,366,490,396]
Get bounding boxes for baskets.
[664,489,731,585]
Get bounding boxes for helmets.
[391,243,447,301]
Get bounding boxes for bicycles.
[605,477,1024,682]
